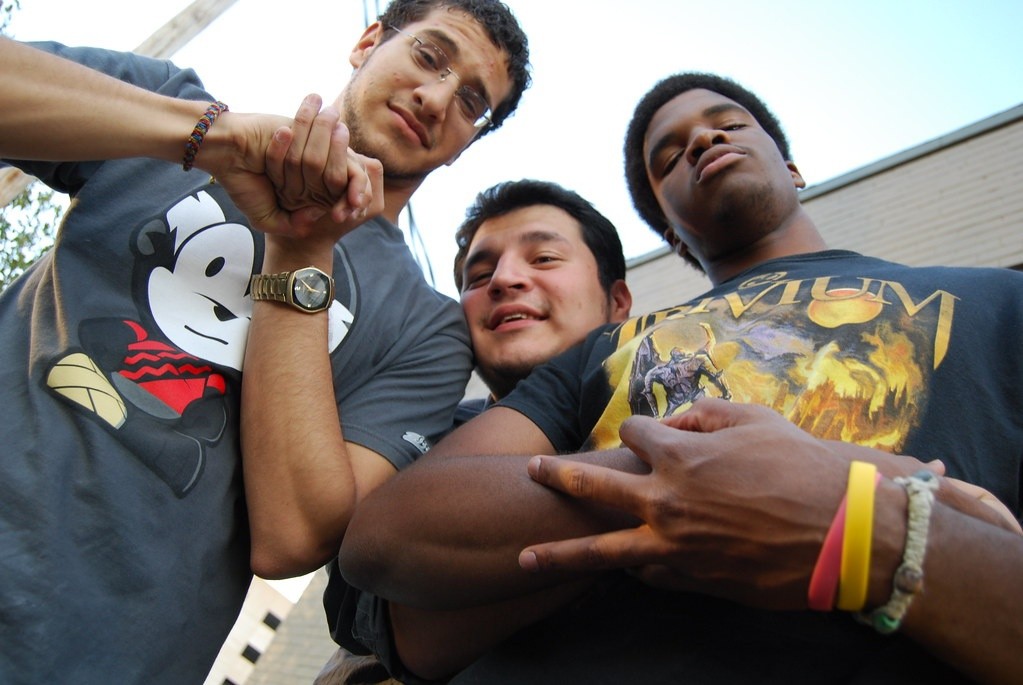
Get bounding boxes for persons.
[338,74,1023,685]
[0,0,529,685]
[313,179,633,685]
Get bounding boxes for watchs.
[248,263,336,314]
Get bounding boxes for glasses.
[387,22,495,131]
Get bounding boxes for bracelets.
[805,459,879,611]
[869,470,940,632]
[180,101,228,173]
